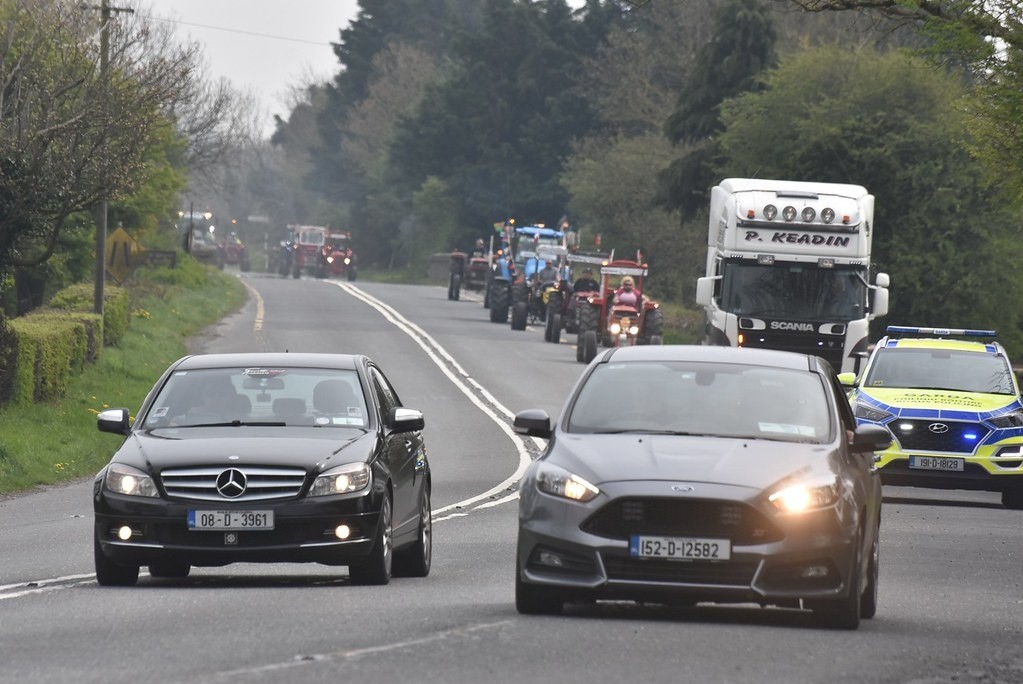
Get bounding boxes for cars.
[509,346,892,631]
[87,351,433,588]
[837,325,1023,510]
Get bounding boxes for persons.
[614,276,649,304]
[573,268,599,291]
[471,238,487,257]
[171,372,236,427]
[538,259,561,281]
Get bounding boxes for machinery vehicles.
[277,224,358,282]
[448,248,497,302]
[177,212,251,272]
[572,260,667,364]
[482,219,615,344]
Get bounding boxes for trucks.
[694,177,892,379]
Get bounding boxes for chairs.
[311,380,354,415]
